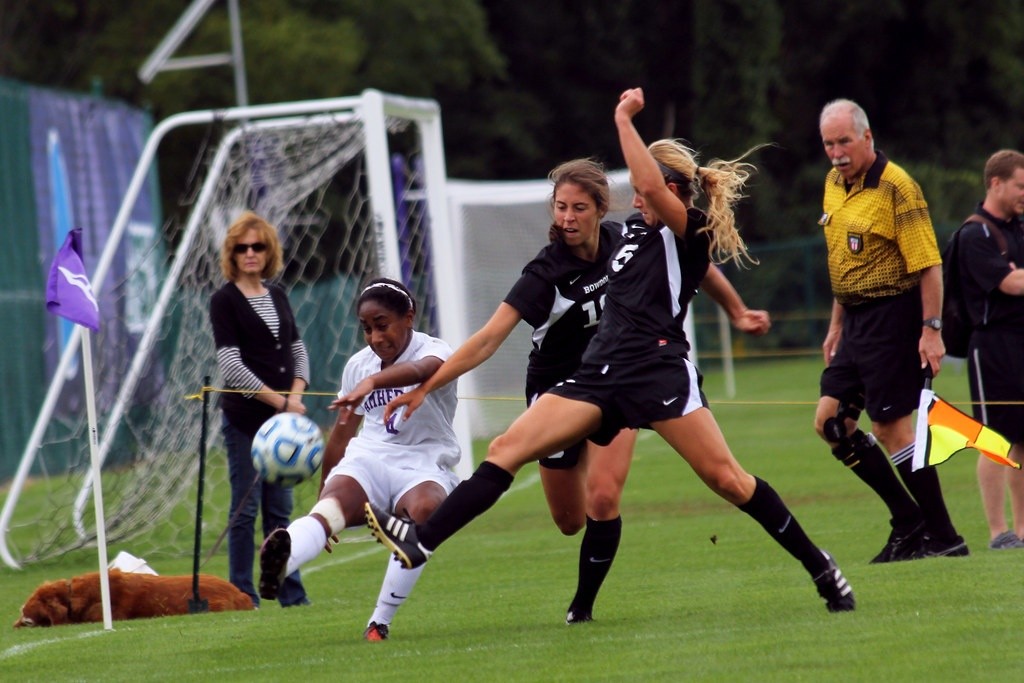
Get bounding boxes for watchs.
[923,317,942,330]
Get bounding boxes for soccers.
[250,411,326,487]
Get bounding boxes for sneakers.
[258,527,291,601]
[868,519,935,563]
[363,622,390,642]
[912,536,970,560]
[364,502,428,570]
[989,531,1024,550]
[812,549,855,612]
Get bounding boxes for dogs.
[14,568,253,628]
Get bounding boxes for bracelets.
[278,397,289,412]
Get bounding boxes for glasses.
[234,242,268,253]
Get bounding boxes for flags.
[47,230,97,331]
[912,389,1021,473]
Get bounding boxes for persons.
[365,88,858,612]
[208,222,313,608]
[384,157,638,625]
[956,150,1024,548]
[260,278,462,644]
[812,100,970,563]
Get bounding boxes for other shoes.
[565,603,593,624]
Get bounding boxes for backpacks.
[940,212,1010,359]
